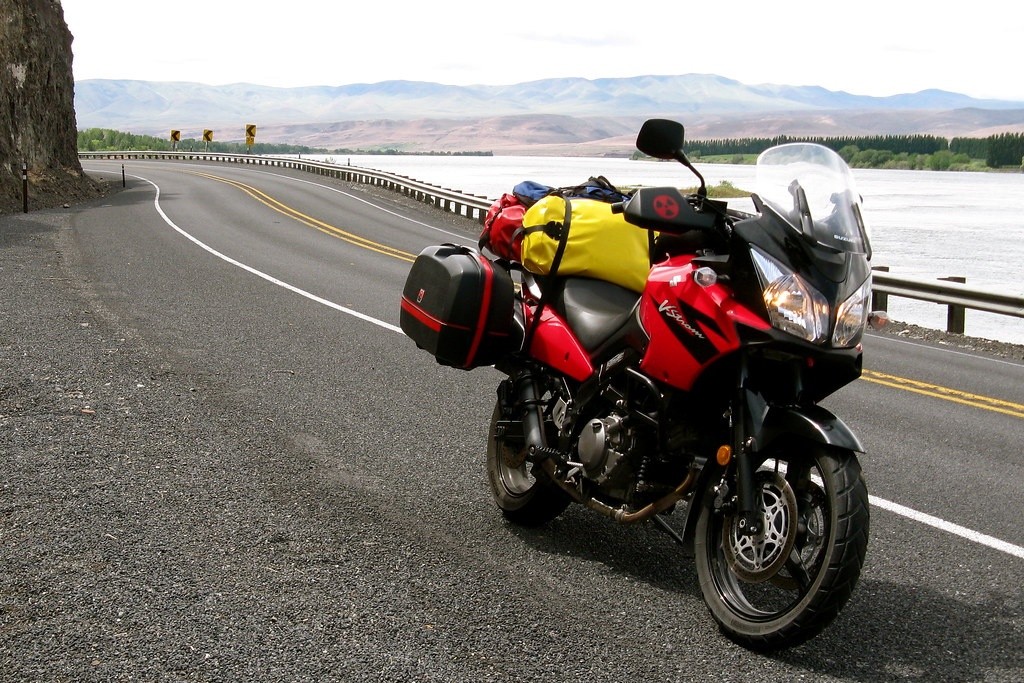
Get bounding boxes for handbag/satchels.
[478,194,530,263]
[509,193,660,294]
[514,175,638,208]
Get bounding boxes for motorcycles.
[401,119,893,660]
